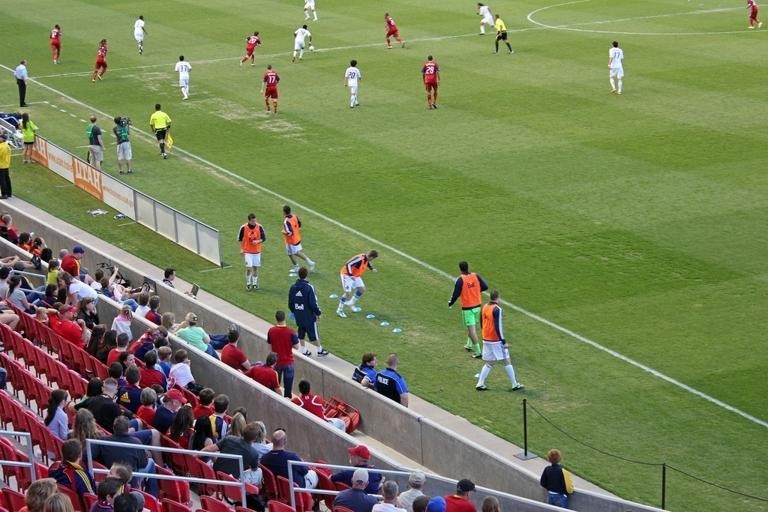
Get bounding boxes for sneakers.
[127,169,133,174]
[183,95,188,99]
[400,41,406,48]
[290,267,300,273]
[346,302,356,311]
[337,309,346,318]
[473,351,481,358]
[617,90,622,94]
[433,102,438,109]
[512,384,523,390]
[476,384,488,390]
[120,170,123,173]
[163,153,167,159]
[246,278,251,286]
[303,350,310,356]
[309,263,316,272]
[350,103,354,107]
[611,88,616,92]
[355,102,359,106]
[464,343,471,349]
[252,279,258,288]
[318,349,329,357]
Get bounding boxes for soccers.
[309,46,315,51]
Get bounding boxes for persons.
[20,113,39,163]
[443,479,477,512]
[111,305,133,345]
[133,16,147,55]
[41,248,54,262]
[89,479,124,512]
[43,492,74,512]
[136,387,157,426]
[290,380,346,432]
[48,439,98,512]
[68,408,105,461]
[151,384,165,403]
[113,366,141,412]
[266,311,301,399]
[92,39,108,82]
[99,278,139,312]
[134,293,151,318]
[608,41,624,95]
[371,480,406,512]
[137,350,166,390]
[156,346,171,380]
[58,271,99,313]
[163,268,194,297]
[18,478,57,512]
[0,302,19,329]
[166,405,193,448]
[188,415,218,467]
[259,429,318,489]
[221,330,252,372]
[61,245,94,287]
[492,15,514,54]
[75,377,103,406]
[330,445,384,495]
[78,295,106,332]
[237,213,268,291]
[476,3,495,35]
[476,290,525,390]
[30,237,47,257]
[344,60,361,108]
[175,312,221,360]
[540,449,574,508]
[97,331,118,362]
[0,134,12,199]
[337,249,378,318]
[100,362,123,398]
[145,295,162,324]
[14,59,27,107]
[280,205,316,275]
[374,353,409,408]
[448,261,489,358]
[303,0,318,22]
[86,116,105,170]
[91,267,149,297]
[288,267,329,357]
[83,416,159,497]
[333,469,378,512]
[396,469,428,512]
[0,266,47,303]
[193,421,263,493]
[291,25,312,62]
[150,104,172,159]
[233,407,247,425]
[5,275,46,318]
[58,249,90,274]
[35,306,57,327]
[106,462,132,490]
[107,334,129,367]
[745,0,763,29]
[1,215,19,243]
[128,325,170,357]
[50,25,62,65]
[193,388,215,419]
[260,65,280,116]
[118,350,136,375]
[86,324,105,356]
[240,32,261,66]
[227,412,246,437]
[44,389,71,438]
[207,394,233,441]
[45,285,59,305]
[68,379,163,468]
[53,305,92,349]
[352,352,378,389]
[161,311,240,349]
[412,495,431,512]
[252,421,273,463]
[384,13,406,49]
[0,254,41,271]
[112,117,132,174]
[114,492,145,512]
[17,232,30,251]
[421,55,440,109]
[168,349,204,394]
[244,352,284,396]
[47,260,60,283]
[152,390,187,435]
[175,55,192,99]
[482,496,501,512]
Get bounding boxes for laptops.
[188,282,200,298]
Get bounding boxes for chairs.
[0,437,15,487]
[83,493,98,512]
[0,352,10,382]
[161,434,185,474]
[259,464,278,500]
[24,337,35,371]
[10,359,22,398]
[217,471,242,502]
[267,500,297,512]
[312,468,337,490]
[56,485,83,512]
[46,354,55,388]
[236,506,258,512]
[1,389,10,430]
[59,336,71,366]
[1,488,30,512]
[35,379,45,418]
[6,298,13,310]
[133,358,146,368]
[14,305,24,330]
[200,495,235,512]
[35,346,47,379]
[24,410,45,462]
[44,426,64,466]
[36,319,48,349]
[0,322,2,342]
[47,327,59,358]
[11,331,24,361]
[183,387,199,409]
[155,464,193,509]
[0,296,4,301]
[168,383,182,391]
[295,492,312,512]
[195,509,210,512]
[14,448,49,495]
[96,425,113,436]
[69,369,79,405]
[21,369,36,408]
[162,498,192,512]
[93,356,108,379]
[9,398,25,431]
[56,361,68,391]
[184,453,216,496]
[3,324,12,355]
[93,461,109,482]
[130,488,161,512]
[80,376,89,396]
[245,482,259,495]
[70,342,81,371]
[137,417,152,430]
[332,506,355,512]
[224,414,234,423]
[336,481,351,491]
[276,475,299,508]
[23,313,37,342]
[81,349,93,378]
[45,387,52,409]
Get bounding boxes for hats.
[352,468,369,484]
[59,304,73,313]
[409,469,426,485]
[458,480,477,493]
[163,389,188,405]
[348,446,370,459]
[73,246,84,254]
[189,314,198,323]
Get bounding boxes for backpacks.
[234,491,267,512]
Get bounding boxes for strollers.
[96,262,133,290]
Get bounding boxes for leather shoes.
[0,194,8,199]
